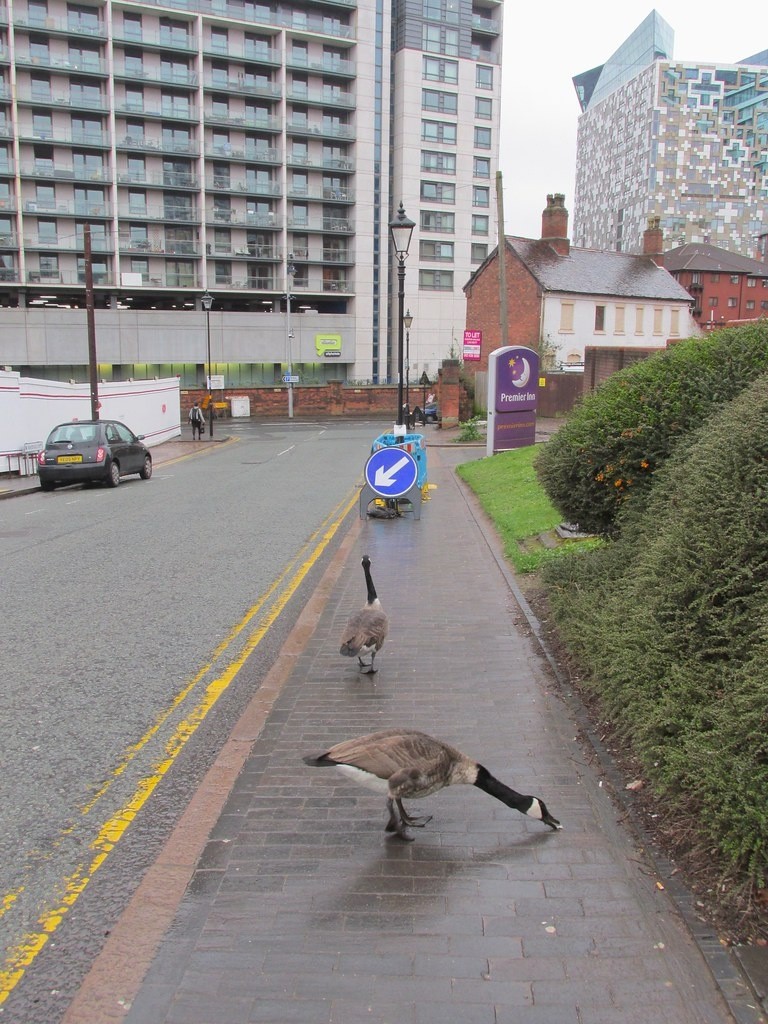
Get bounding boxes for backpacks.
[191,407,200,422]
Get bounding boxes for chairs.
[69,430,84,440]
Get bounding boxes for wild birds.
[339,555,388,675]
[303,727,562,842]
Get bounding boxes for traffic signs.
[281,376,299,382]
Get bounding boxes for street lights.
[401,310,414,431]
[200,289,214,436]
[388,200,415,436]
[286,265,297,418]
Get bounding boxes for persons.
[189,401,205,440]
[402,400,411,425]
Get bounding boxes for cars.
[36,420,152,491]
[420,401,438,423]
